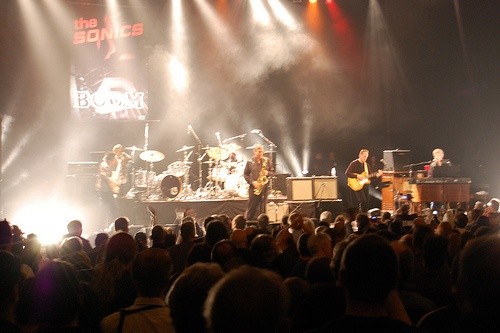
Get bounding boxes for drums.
[209,159,250,197]
[132,159,191,200]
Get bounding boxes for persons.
[429,149,454,210]
[99,162,117,228]
[229,152,237,174]
[103,144,131,171]
[244,144,274,221]
[0,198,500,333]
[345,149,383,213]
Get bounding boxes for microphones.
[188,125,191,134]
[422,160,432,164]
[215,131,221,145]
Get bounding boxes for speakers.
[286,176,338,200]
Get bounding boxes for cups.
[330,168,336,177]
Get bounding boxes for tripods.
[124,124,228,201]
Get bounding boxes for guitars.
[348,168,385,192]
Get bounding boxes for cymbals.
[139,151,165,162]
[175,145,196,152]
[206,148,230,161]
[124,146,144,151]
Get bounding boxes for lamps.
[302,169,310,177]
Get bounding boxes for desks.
[409,183,471,216]
[284,199,345,228]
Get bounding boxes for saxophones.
[253,156,269,196]
